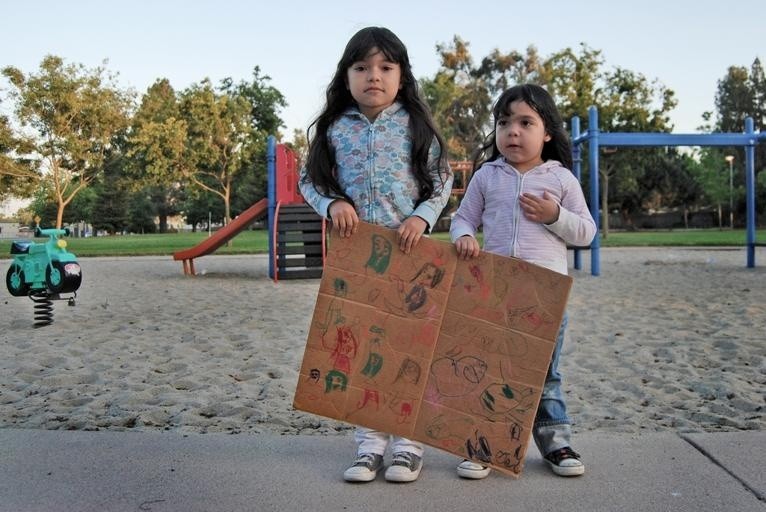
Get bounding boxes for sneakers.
[342,454,386,482]
[455,458,492,480]
[546,446,586,477]
[385,452,424,484]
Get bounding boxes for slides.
[174,197,269,260]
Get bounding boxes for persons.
[295,25,457,485]
[444,83,599,481]
